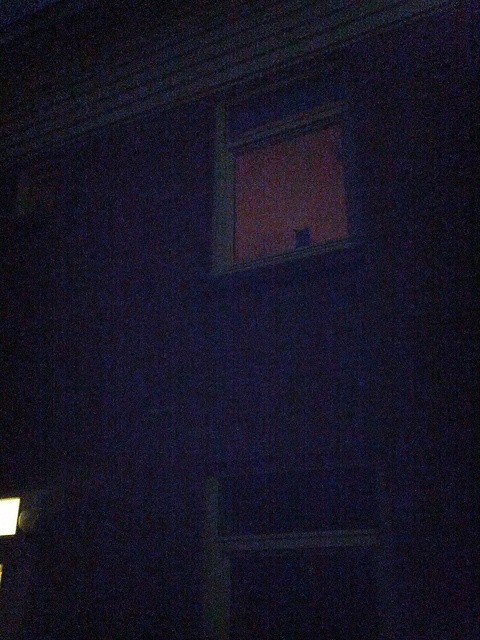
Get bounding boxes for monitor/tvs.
[1,494,22,536]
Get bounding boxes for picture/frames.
[199,525,390,638]
[211,101,357,278]
